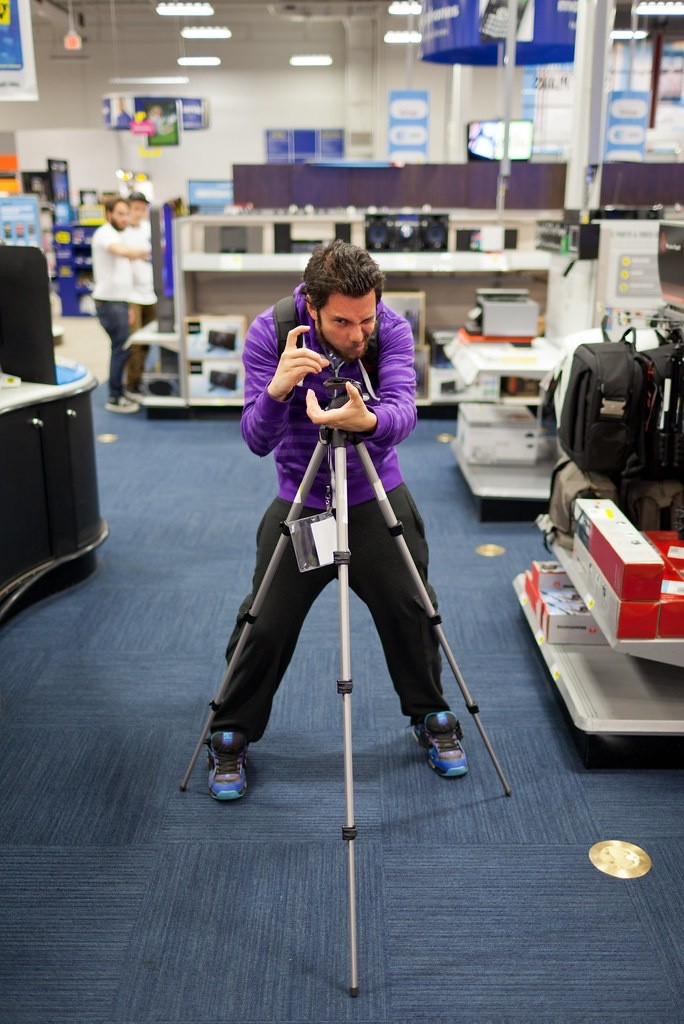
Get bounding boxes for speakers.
[366,214,448,253]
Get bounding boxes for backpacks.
[538,312,683,562]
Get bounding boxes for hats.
[128,192,150,202]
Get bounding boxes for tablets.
[220,226,247,254]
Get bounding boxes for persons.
[107,95,178,139]
[119,191,159,399]
[89,197,150,414]
[204,236,470,801]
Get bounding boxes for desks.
[0,358,111,634]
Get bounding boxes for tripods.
[178,373,512,1000]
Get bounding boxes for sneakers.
[105,394,141,415]
[202,728,249,802]
[413,708,469,779]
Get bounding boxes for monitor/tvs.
[468,119,533,161]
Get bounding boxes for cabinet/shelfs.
[118,201,684,739]
[54,223,101,318]
